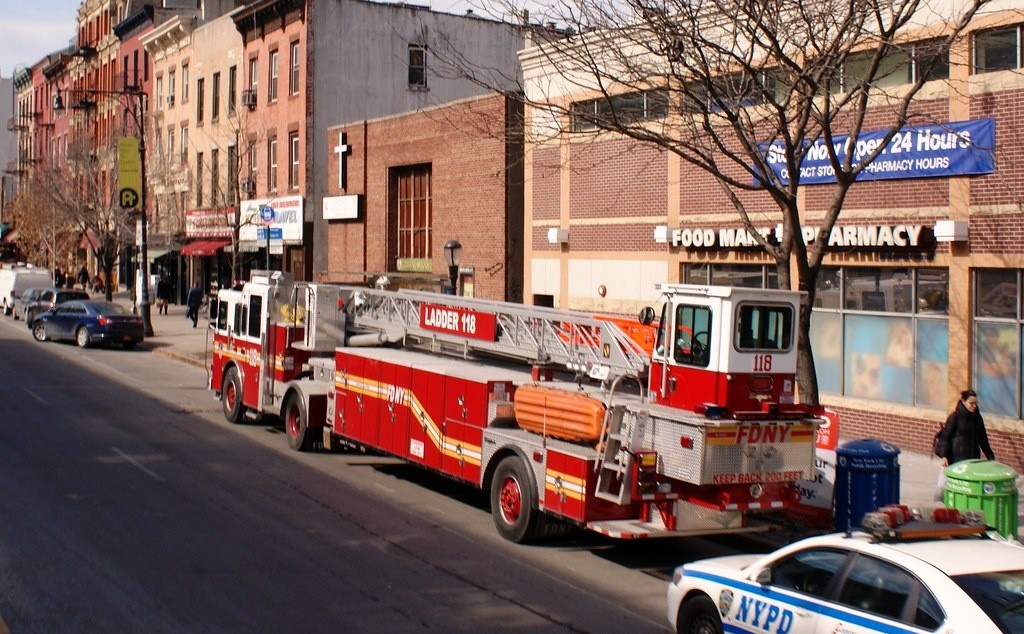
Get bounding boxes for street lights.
[53,86,156,336]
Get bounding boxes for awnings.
[180,241,231,256]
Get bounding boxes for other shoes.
[193,321,197,327]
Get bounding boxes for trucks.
[1,261,52,316]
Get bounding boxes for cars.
[665,501,1024,634]
[32,298,145,349]
[12,287,90,328]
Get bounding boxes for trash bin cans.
[944,459,1018,541]
[829,439,902,534]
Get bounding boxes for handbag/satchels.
[932,464,947,503]
[155,298,164,306]
[185,309,189,317]
[931,421,945,460]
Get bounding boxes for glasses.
[968,402,979,404]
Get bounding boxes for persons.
[934,390,996,468]
[77,266,92,290]
[50,265,67,282]
[156,271,172,316]
[185,280,204,329]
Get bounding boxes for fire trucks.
[204,267,826,546]
